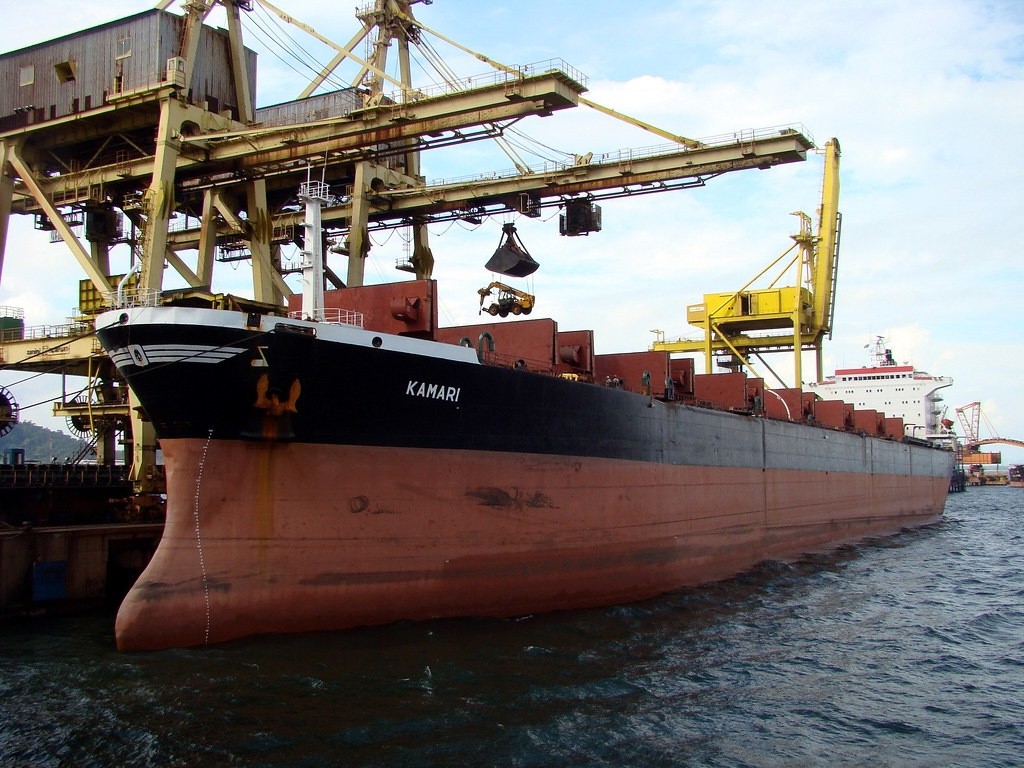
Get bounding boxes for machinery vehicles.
[478,282,534,318]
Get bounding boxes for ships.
[94,292,961,655]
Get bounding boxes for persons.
[748,392,761,412]
[606,375,623,387]
[807,414,816,427]
